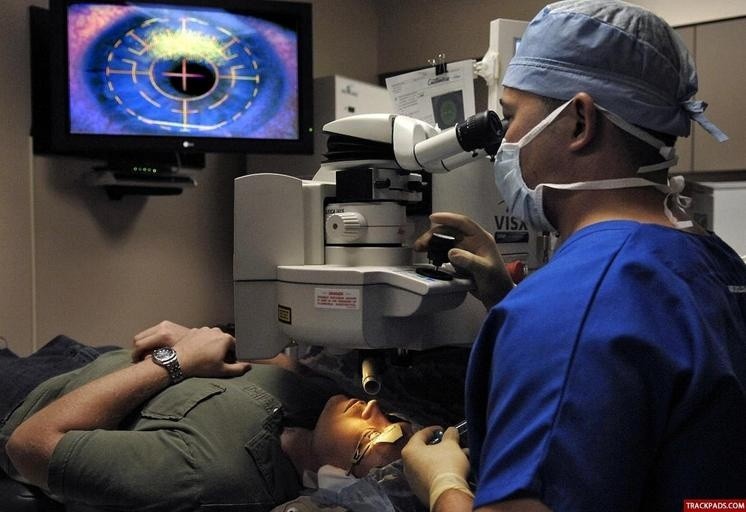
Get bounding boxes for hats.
[502,1,699,138]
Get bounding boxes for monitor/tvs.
[48,0,313,156]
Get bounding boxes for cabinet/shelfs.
[667,14,746,175]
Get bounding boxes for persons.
[0,320,416,512]
[400,0,746,512]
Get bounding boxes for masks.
[494,99,574,233]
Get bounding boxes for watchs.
[151,346,184,383]
